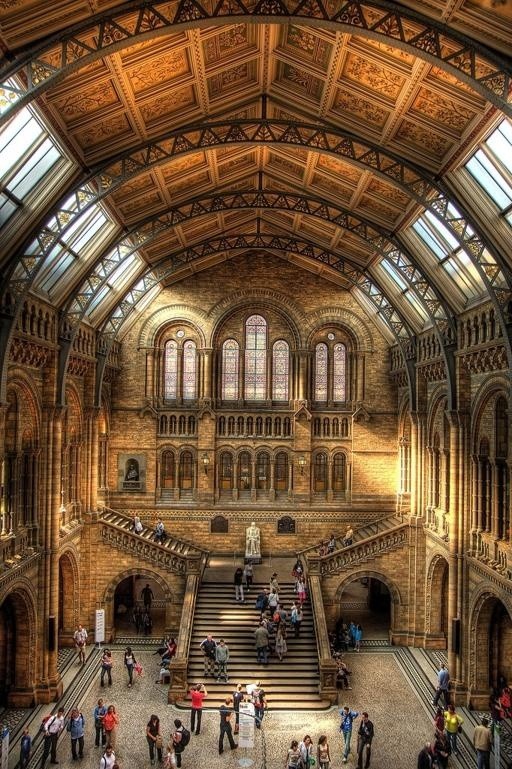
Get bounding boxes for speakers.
[452,618,460,653]
[49,616,56,651]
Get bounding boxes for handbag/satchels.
[310,757,315,765]
[459,726,462,733]
[292,614,297,624]
[256,600,264,608]
[156,735,163,749]
[273,613,280,623]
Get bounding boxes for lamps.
[298,455,307,477]
[201,451,210,475]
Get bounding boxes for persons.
[327,534,335,554]
[126,464,138,481]
[153,518,165,545]
[132,512,140,534]
[246,521,260,555]
[16,560,512,769]
[344,525,353,546]
[318,540,326,556]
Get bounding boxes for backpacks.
[179,727,190,746]
[266,621,275,634]
[252,690,261,708]
[39,717,56,732]
